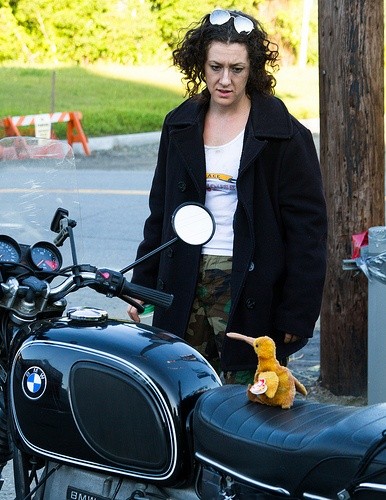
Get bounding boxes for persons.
[125,10,329,387]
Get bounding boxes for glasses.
[210,9,254,36]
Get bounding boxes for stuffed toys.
[226,332,308,408]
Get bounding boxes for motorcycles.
[0,135,386,500]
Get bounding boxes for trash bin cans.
[342,227,386,406]
